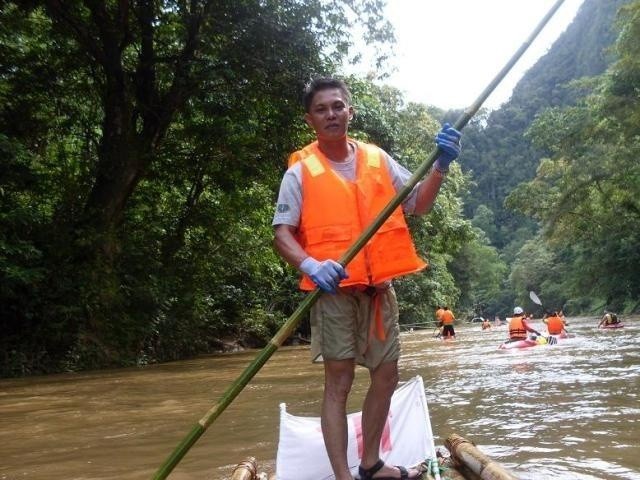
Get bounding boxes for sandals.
[354,459,424,480]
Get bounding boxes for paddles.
[480,316,485,321]
[529,290,576,338]
[505,317,557,345]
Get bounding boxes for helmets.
[513,307,523,315]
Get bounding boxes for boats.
[541,328,576,339]
[496,335,547,350]
[227,432,516,480]
[599,321,625,329]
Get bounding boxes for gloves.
[433,122,464,175]
[297,255,351,296]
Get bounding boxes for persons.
[556,309,566,325]
[440,307,457,337]
[481,319,490,330]
[269,76,464,480]
[542,311,565,336]
[597,310,618,328]
[508,306,543,341]
[434,305,444,328]
